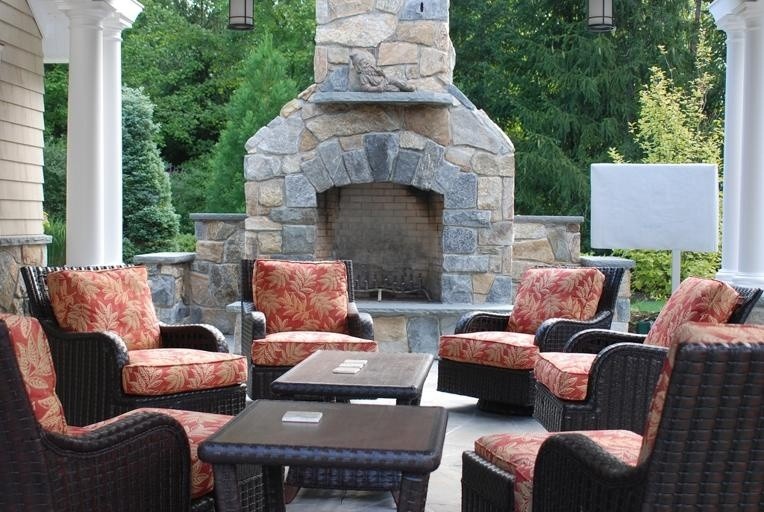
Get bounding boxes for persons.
[348,51,416,92]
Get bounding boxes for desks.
[197,398,450,512]
[270,348,435,406]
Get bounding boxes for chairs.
[238,260,375,405]
[435,263,629,418]
[20,262,248,429]
[530,275,764,436]
[461,338,764,510]
[0,311,237,512]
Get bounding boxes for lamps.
[587,0,615,31]
[227,0,255,31]
